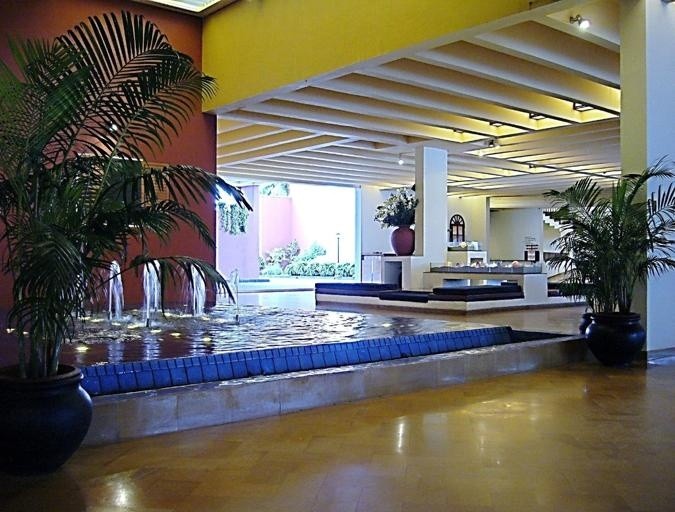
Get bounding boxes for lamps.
[398,153,404,164]
[570,14,591,30]
[488,140,494,147]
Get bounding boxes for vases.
[391,227,416,257]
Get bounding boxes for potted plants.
[1,9,253,474]
[541,153,675,366]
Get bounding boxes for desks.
[449,249,488,268]
[423,269,548,299]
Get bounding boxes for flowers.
[374,187,420,228]
